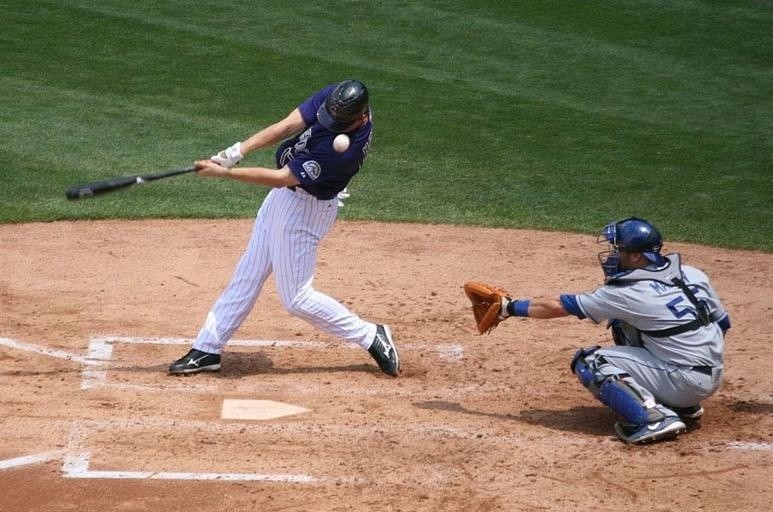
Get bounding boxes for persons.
[497,218,729,445]
[166,80,399,378]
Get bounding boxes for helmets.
[317,79,368,133]
[597,218,666,276]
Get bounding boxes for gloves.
[337,187,350,207]
[211,141,244,169]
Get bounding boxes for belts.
[287,185,334,200]
[692,366,711,375]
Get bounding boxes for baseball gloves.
[464,280,512,334]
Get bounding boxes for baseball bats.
[65,166,201,201]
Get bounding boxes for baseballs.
[331,134,351,152]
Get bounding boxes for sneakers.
[169,348,221,373]
[663,404,704,418]
[614,403,686,444]
[368,324,400,376]
